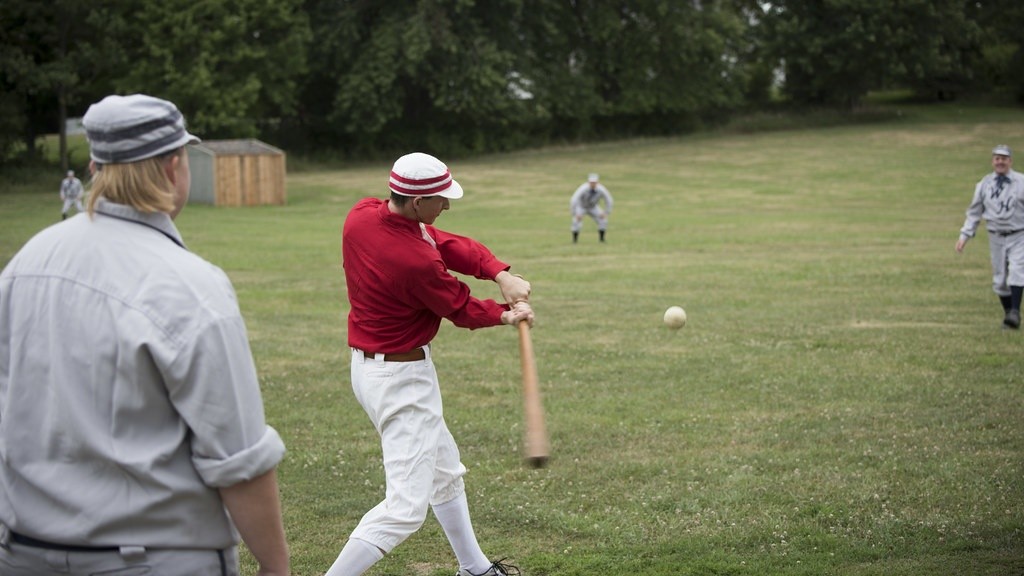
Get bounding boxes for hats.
[588,174,599,183]
[82,94,201,164]
[389,152,464,199]
[992,144,1012,157]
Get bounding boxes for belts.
[363,348,425,361]
[989,230,1016,237]
[6,532,118,553]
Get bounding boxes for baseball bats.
[510,270,554,469]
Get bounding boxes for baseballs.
[661,304,688,331]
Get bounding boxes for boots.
[1003,286,1024,329]
[573,231,578,243]
[599,229,605,242]
[1000,295,1010,315]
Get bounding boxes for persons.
[569,172,613,244]
[60,169,84,221]
[323,152,536,576]
[0,92,294,576]
[954,143,1024,329]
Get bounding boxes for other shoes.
[456,557,520,576]
